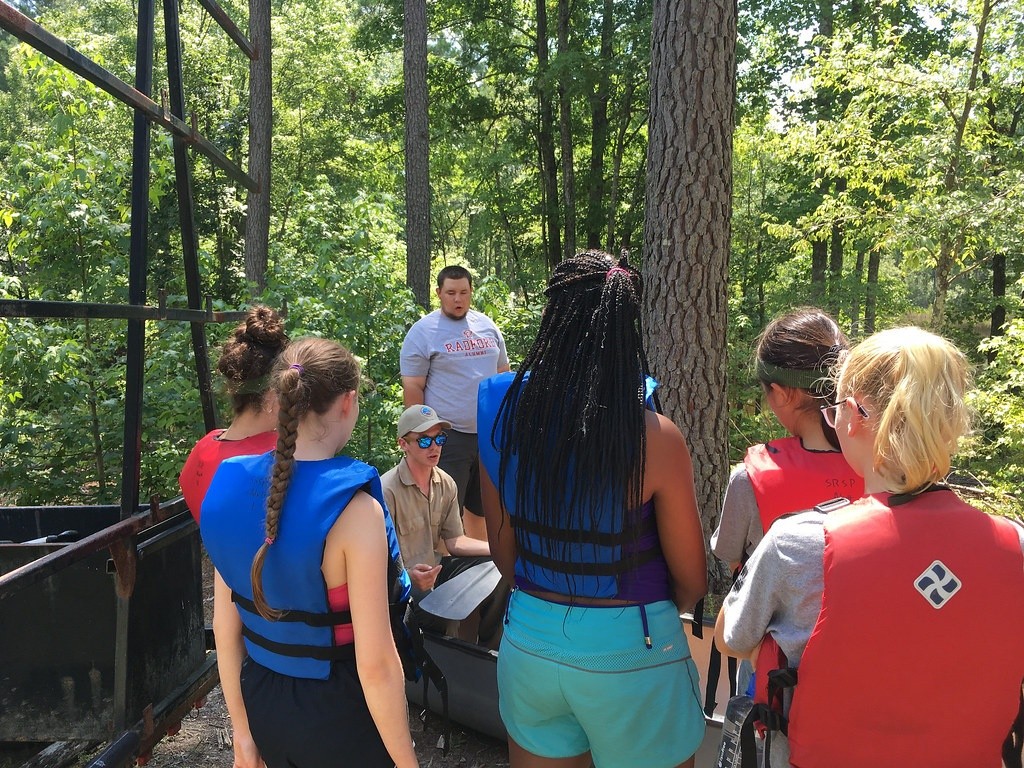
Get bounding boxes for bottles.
[714,673,759,768]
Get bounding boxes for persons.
[380,404,510,651]
[400,266,509,540]
[478,248,709,768]
[709,307,869,768]
[715,327,1024,768]
[199,338,422,768]
[178,307,295,525]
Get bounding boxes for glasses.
[403,431,448,448]
[821,398,868,428]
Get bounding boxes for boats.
[405,613,760,768]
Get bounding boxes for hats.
[398,404,452,440]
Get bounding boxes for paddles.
[415,559,501,623]
